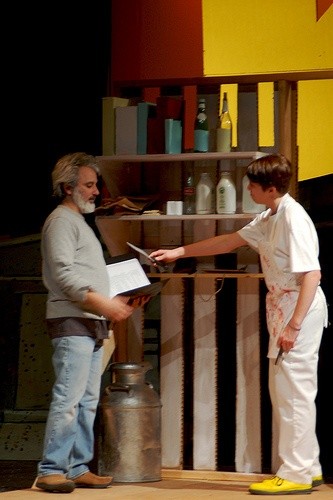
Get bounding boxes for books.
[106,258,170,305]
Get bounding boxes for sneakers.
[312,473,323,488]
[35,474,74,493]
[66,471,113,488]
[248,476,312,494]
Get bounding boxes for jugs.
[90,361,164,485]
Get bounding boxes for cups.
[166,201,183,216]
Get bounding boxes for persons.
[152,153,327,494]
[36,152,153,493]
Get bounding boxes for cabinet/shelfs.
[95,151,273,277]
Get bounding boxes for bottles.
[216,93,232,153]
[216,173,236,214]
[196,172,214,215]
[182,172,196,214]
[241,175,266,214]
[193,99,209,153]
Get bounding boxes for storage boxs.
[103,96,182,156]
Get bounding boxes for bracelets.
[287,322,302,331]
